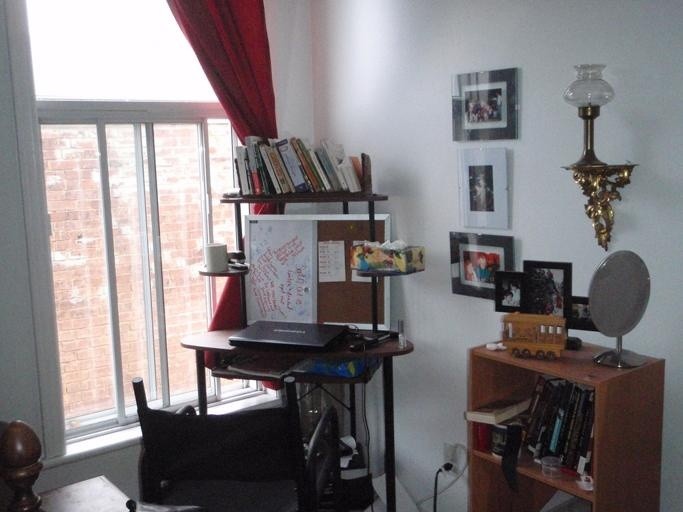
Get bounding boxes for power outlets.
[442,444,458,474]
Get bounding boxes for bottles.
[538,325,546,343]
[542,457,560,477]
[547,325,554,343]
[556,327,562,344]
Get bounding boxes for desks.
[2,473,150,511]
[182,329,414,511]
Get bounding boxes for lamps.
[562,62,637,254]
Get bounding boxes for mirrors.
[587,250,650,369]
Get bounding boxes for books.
[227,353,302,378]
[463,365,595,489]
[233,133,362,194]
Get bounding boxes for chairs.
[132,377,341,512]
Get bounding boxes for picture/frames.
[572,296,598,332]
[450,230,514,300]
[494,270,523,313]
[522,259,572,330]
[457,147,512,231]
[452,68,517,141]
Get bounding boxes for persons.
[502,280,520,307]
[474,253,492,283]
[464,88,502,125]
[464,256,477,281]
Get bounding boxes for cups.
[205,243,227,271]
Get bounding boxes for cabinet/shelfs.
[467,349,664,511]
[200,150,389,334]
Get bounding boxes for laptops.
[227,320,349,352]
